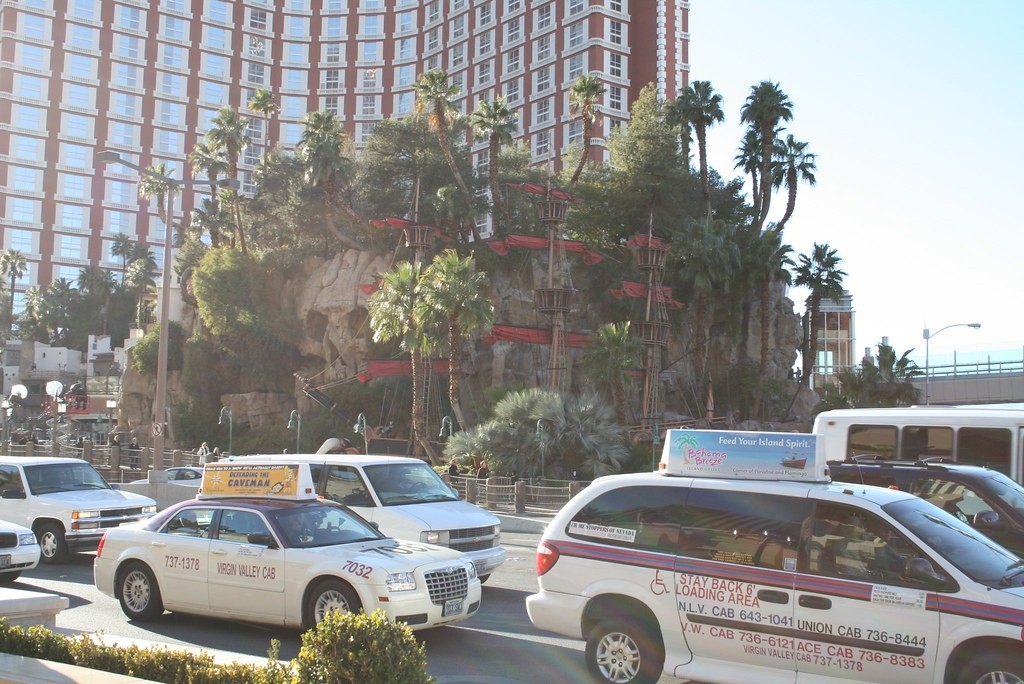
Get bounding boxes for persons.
[476,462,490,503]
[383,468,422,496]
[214,447,219,456]
[871,524,915,573]
[130,437,139,470]
[19,435,39,456]
[789,367,800,382]
[111,435,121,467]
[449,460,460,488]
[53,470,80,485]
[944,487,971,524]
[197,443,209,467]
[509,471,518,504]
[76,437,83,458]
[285,513,334,545]
[284,449,288,454]
[388,421,395,438]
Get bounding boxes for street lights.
[96,150,177,470]
[353,412,369,457]
[921,322,984,406]
[218,405,233,460]
[286,409,301,454]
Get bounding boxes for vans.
[809,405,1024,495]
[217,451,505,583]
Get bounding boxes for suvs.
[820,450,1024,560]
[0,453,160,566]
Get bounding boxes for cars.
[130,466,204,491]
[91,462,483,646]
[525,430,1023,684]
[0,520,43,585]
